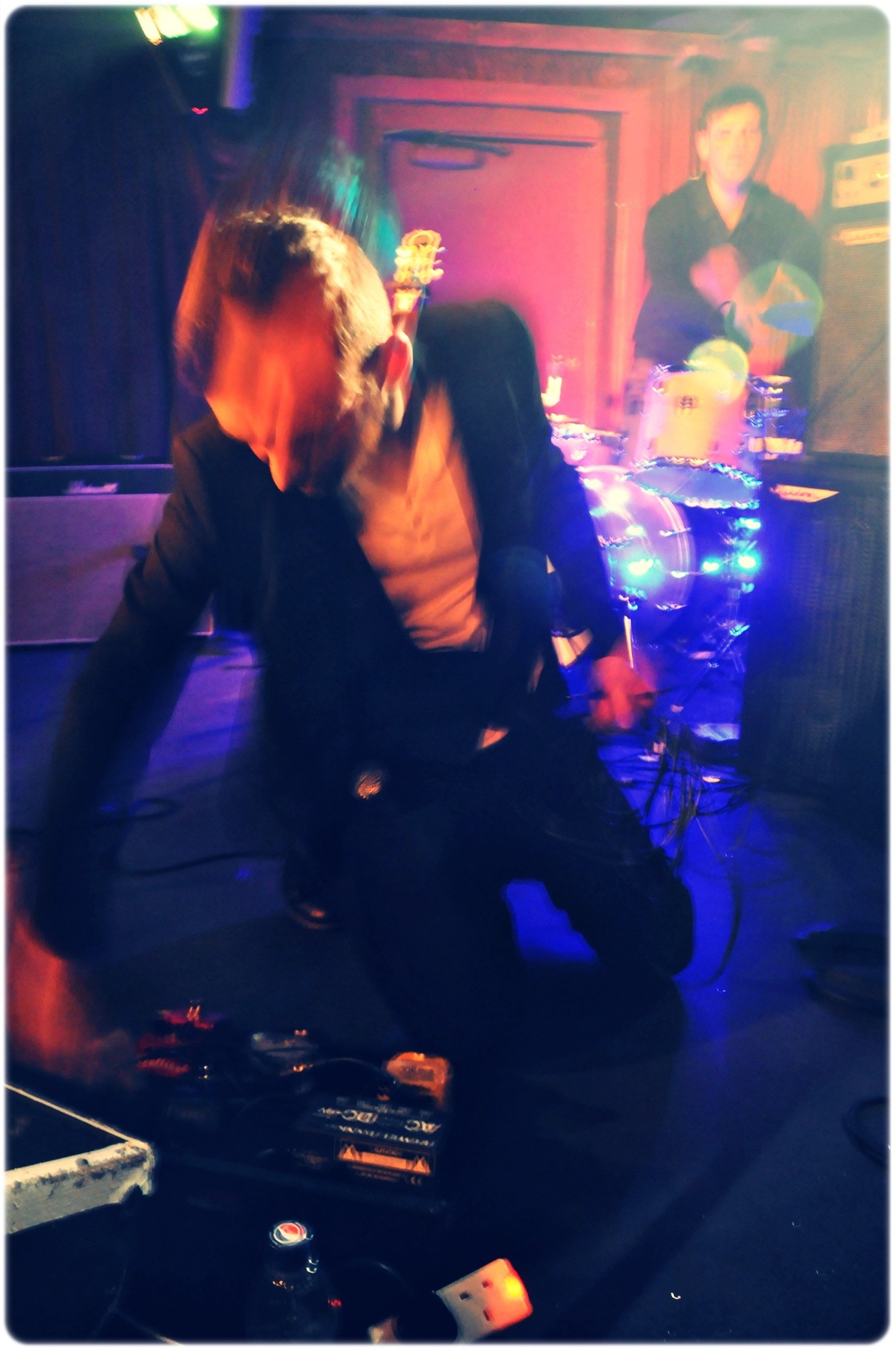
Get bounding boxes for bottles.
[245,1221,341,1343]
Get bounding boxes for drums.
[569,465,696,638]
[542,420,625,471]
[628,359,771,513]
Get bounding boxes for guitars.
[394,225,450,317]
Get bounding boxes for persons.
[629,86,822,454]
[6,213,696,1047]
